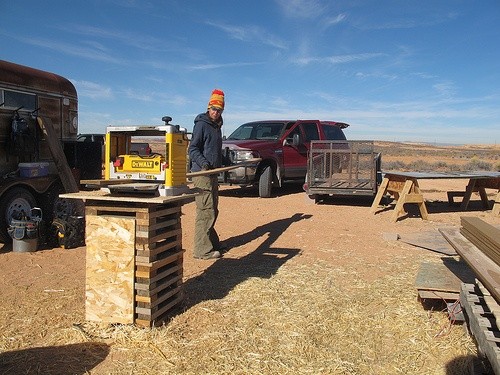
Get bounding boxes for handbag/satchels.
[49,217,81,248]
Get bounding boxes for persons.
[188,89,234,260]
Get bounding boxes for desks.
[59,191,200,328]
[371,169,500,222]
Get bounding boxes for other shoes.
[214,242,227,251]
[193,251,220,258]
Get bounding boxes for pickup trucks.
[78,133,151,191]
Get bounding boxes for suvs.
[217,119,350,198]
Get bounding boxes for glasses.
[211,106,223,114]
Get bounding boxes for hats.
[208,89,224,110]
